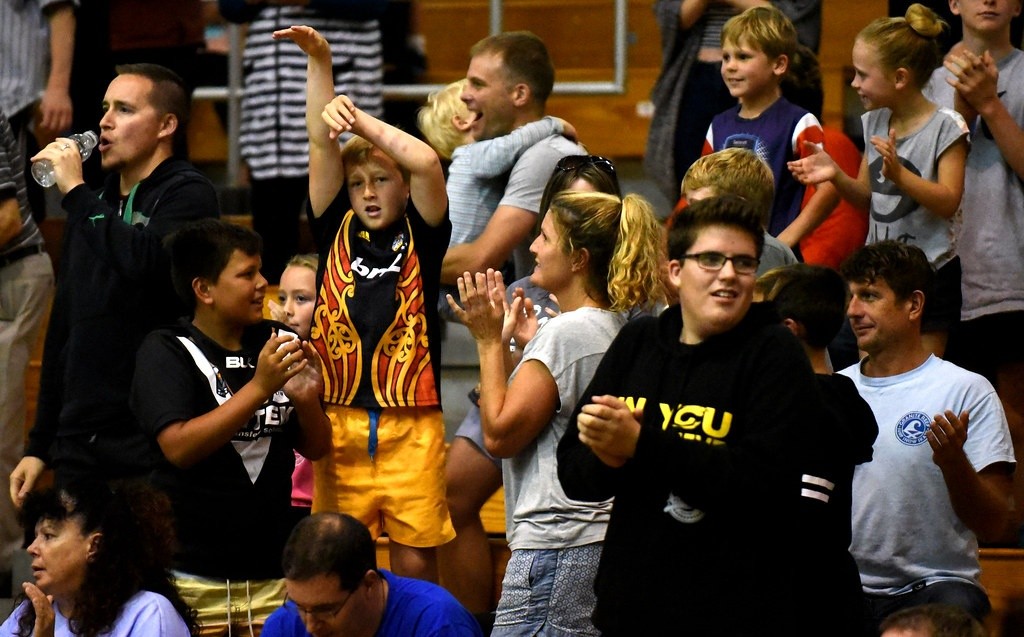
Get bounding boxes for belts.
[0,243,47,268]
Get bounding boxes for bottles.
[31,131,99,189]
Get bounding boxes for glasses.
[684,252,760,274]
[555,156,615,175]
[282,574,362,621]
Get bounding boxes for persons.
[0,0,1024,637]
[557,195,820,637]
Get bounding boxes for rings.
[60,143,70,151]
